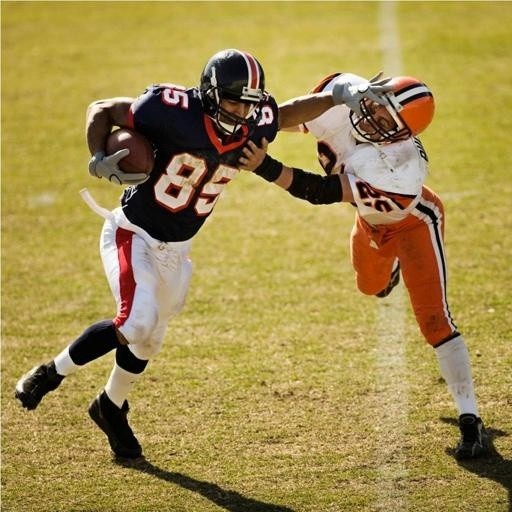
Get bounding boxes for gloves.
[332,72,394,118]
[88,148,151,185]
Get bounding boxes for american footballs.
[107,129,155,174]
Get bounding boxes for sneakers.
[88,388,142,458]
[376,261,400,297]
[454,413,489,458]
[15,359,66,410]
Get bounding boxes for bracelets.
[254,153,286,184]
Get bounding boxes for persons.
[14,50,396,457]
[238,72,494,460]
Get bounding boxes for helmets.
[349,75,434,145]
[198,49,271,145]
